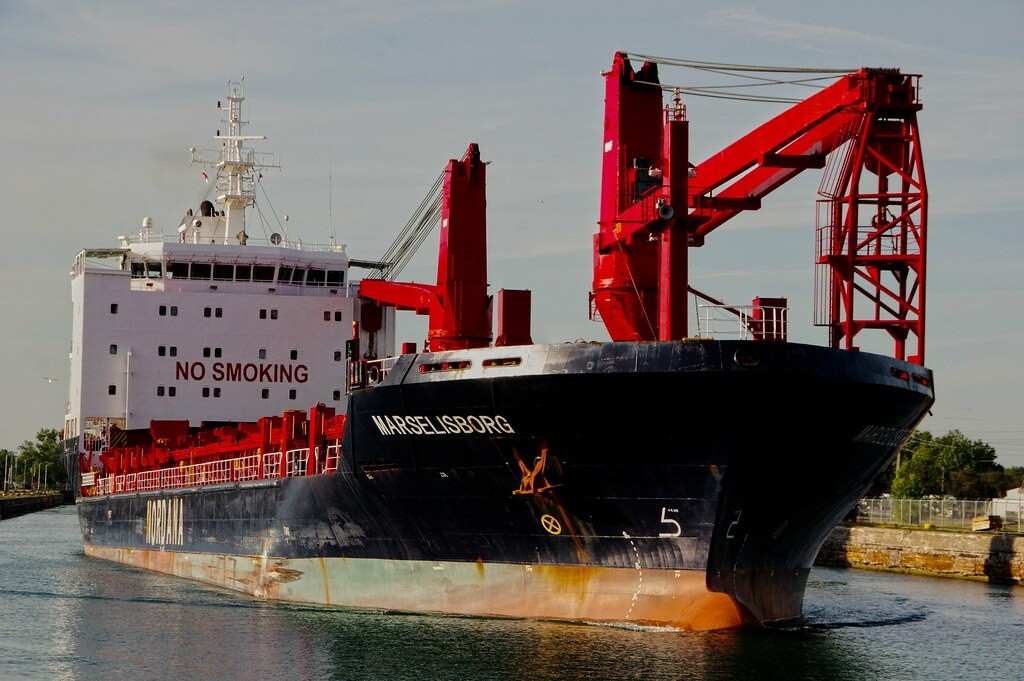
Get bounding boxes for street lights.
[4,450,54,492]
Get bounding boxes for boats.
[60,68,935,633]
